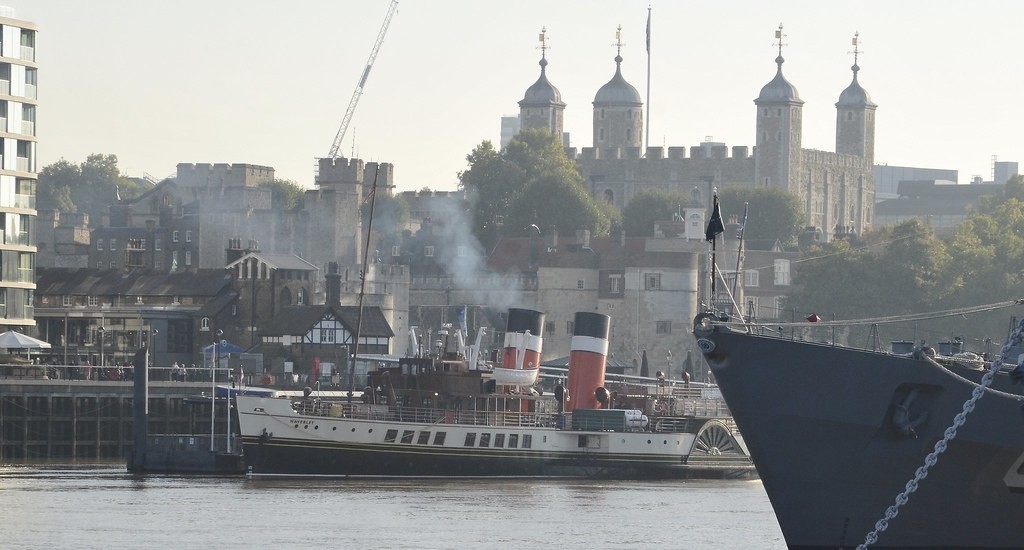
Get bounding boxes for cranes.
[314,0,401,182]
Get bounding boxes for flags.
[706,201,725,242]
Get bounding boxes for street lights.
[151,328,159,380]
[216,329,223,380]
[97,326,106,370]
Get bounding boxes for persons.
[171,362,196,382]
[48,358,151,381]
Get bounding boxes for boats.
[235,168,698,481]
[691,294,1024,550]
